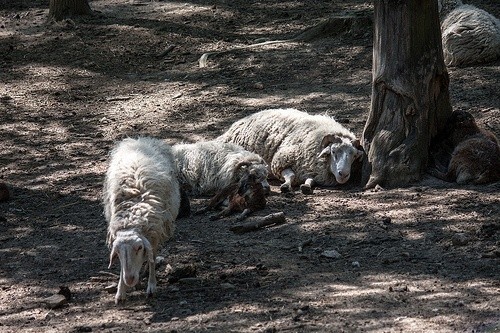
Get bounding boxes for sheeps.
[441,4,500,68]
[423,109,500,186]
[101,132,182,306]
[169,107,364,222]
